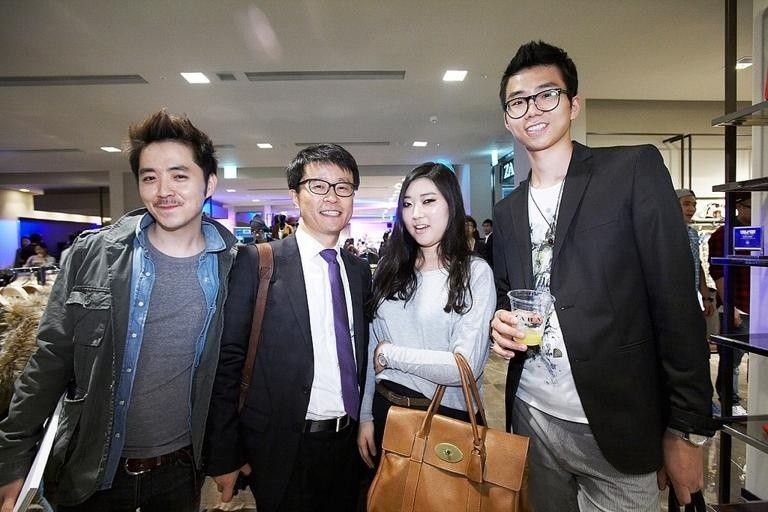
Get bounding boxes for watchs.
[376,342,394,370]
[663,425,710,448]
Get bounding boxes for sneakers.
[732,405,750,424]
[712,402,721,417]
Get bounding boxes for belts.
[302,416,353,435]
[116,446,192,474]
[377,386,433,406]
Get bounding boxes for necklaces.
[527,174,566,245]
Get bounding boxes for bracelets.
[702,295,716,301]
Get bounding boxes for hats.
[675,188,694,197]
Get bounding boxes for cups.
[508,290,555,347]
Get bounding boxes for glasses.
[504,88,569,118]
[740,203,752,209]
[296,178,357,197]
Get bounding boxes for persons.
[0,111,237,512]
[676,187,716,321]
[353,161,498,469]
[488,40,717,512]
[464,216,488,264]
[13,236,45,263]
[204,144,372,512]
[707,191,751,407]
[482,218,494,269]
[24,243,57,268]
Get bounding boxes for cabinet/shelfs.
[689,0,763,510]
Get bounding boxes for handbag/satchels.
[212,244,273,497]
[366,352,532,511]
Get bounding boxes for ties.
[320,248,360,422]
